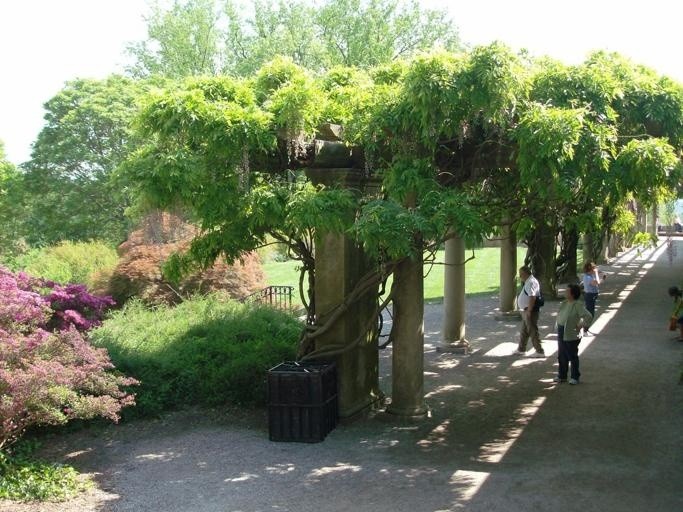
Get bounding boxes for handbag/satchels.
[536,296,544,306]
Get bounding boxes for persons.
[553,282,593,385]
[667,286,683,342]
[582,262,601,338]
[511,267,545,358]
[579,262,608,286]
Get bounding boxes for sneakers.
[513,350,526,354]
[554,377,567,382]
[569,379,579,385]
[530,352,545,357]
[584,330,594,337]
